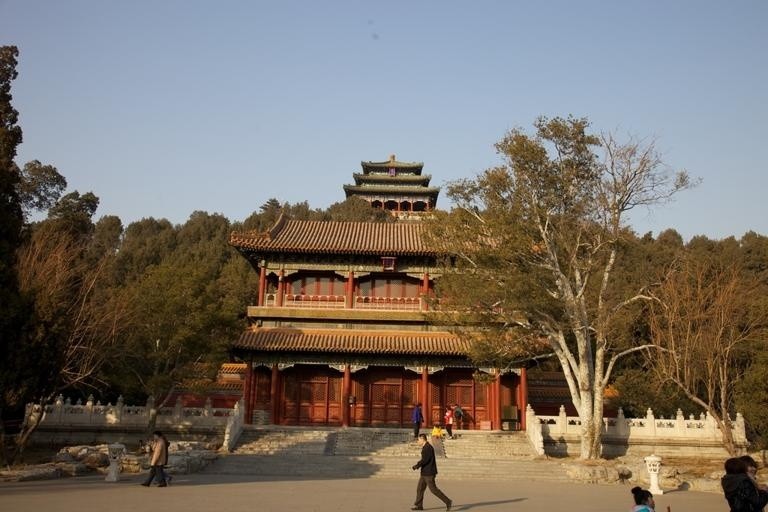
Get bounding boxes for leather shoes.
[445,500,453,512]
[410,505,425,511]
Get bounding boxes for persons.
[140,430,169,487]
[741,455,759,479]
[411,433,453,511]
[150,434,172,485]
[444,406,453,438]
[721,457,768,512]
[413,402,424,437]
[453,404,465,430]
[630,485,658,512]
